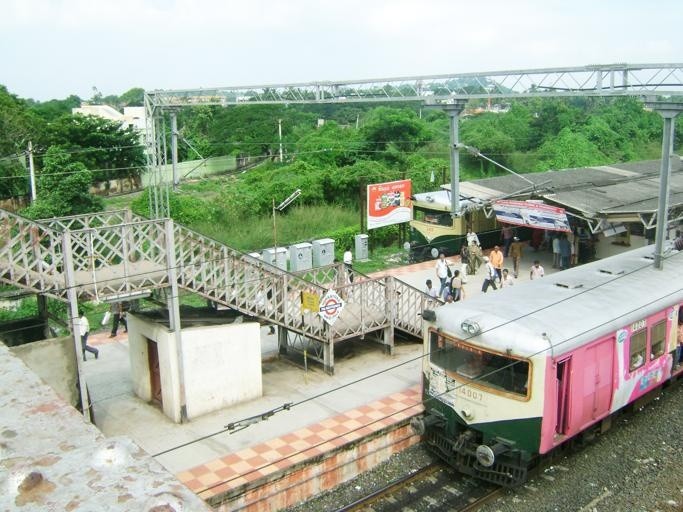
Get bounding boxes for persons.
[343,244,354,284]
[530,261,545,281]
[672,322,679,370]
[631,351,642,368]
[106,300,128,340]
[77,308,99,362]
[558,235,572,270]
[679,319,682,363]
[551,236,561,268]
[422,224,524,310]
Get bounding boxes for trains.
[409,190,534,264]
[410,235,683,487]
